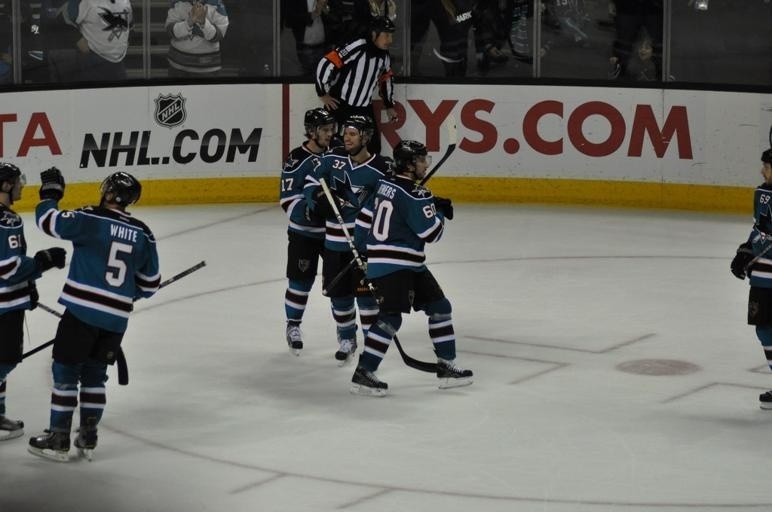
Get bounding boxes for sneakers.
[758,390,772,404]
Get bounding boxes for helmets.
[343,115,375,139]
[0,163,27,191]
[100,170,141,203]
[365,16,397,35]
[304,107,336,126]
[393,139,430,170]
[760,147,772,167]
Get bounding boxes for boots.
[28,429,71,452]
[0,410,25,431]
[436,358,472,378]
[351,366,387,390]
[73,428,98,451]
[285,320,307,350]
[335,338,358,360]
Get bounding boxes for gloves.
[729,243,756,282]
[306,194,341,221]
[38,166,66,200]
[34,247,68,269]
[435,194,454,221]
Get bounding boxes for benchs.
[0,0,772,91]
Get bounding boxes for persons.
[729,148,772,404]
[0,0,135,87]
[303,114,398,360]
[26,168,160,452]
[0,162,67,432]
[279,108,341,349]
[311,13,397,154]
[161,3,709,83]
[352,138,473,390]
